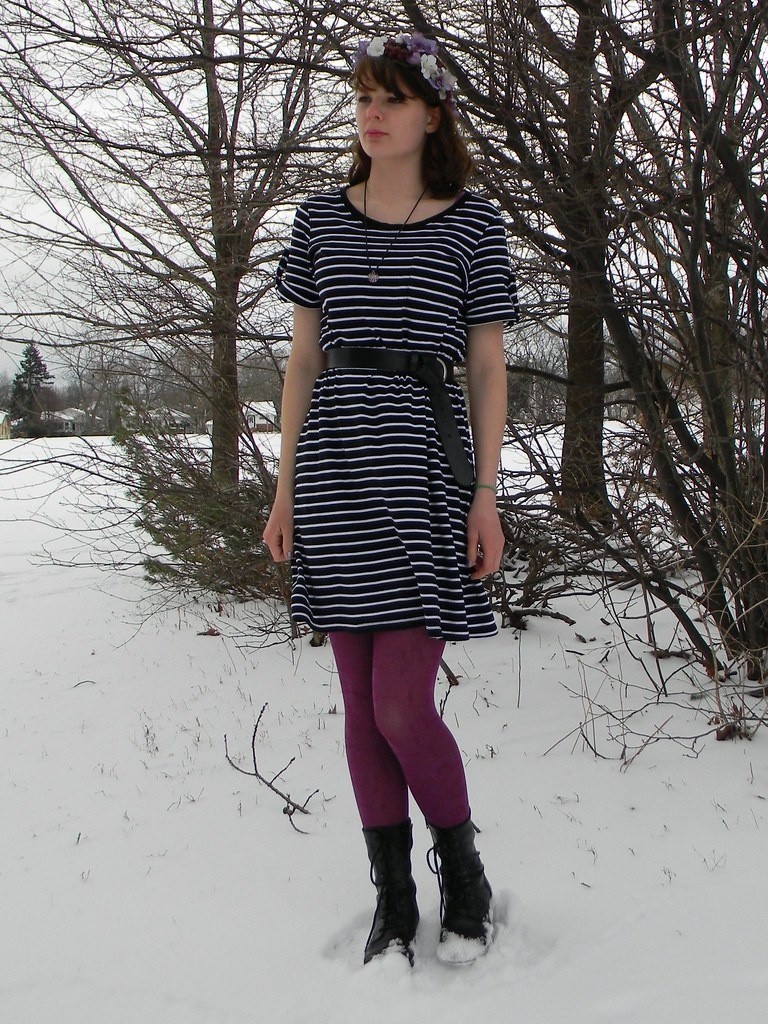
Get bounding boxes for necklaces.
[364,177,429,283]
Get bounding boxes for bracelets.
[476,484,498,494]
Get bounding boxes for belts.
[322,347,473,488]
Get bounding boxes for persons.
[260,34,519,970]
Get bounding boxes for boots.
[426,810,492,966]
[362,819,419,967]
